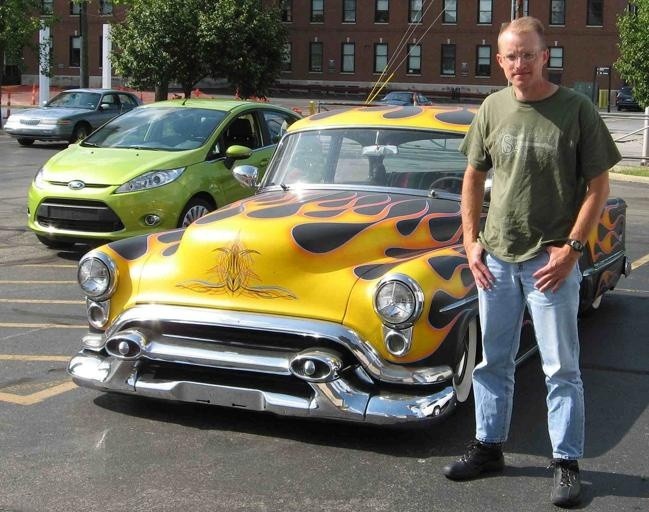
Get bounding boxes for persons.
[442,17,623,506]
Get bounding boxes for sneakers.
[444,441,504,481]
[549,459,579,505]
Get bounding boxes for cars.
[3,89,144,146]
[27,99,305,249]
[374,92,433,106]
[615,86,643,111]
[67,104,631,428]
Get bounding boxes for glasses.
[499,48,541,63]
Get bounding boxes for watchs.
[566,236,585,251]
